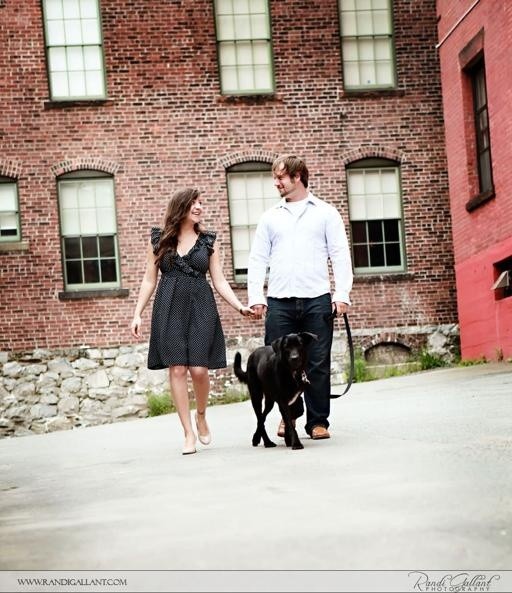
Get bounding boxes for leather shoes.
[310,424,330,439]
[194,413,213,444]
[278,418,295,437]
[181,431,197,454]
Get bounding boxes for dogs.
[233,331,320,450]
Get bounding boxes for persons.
[247,154,354,438]
[131,188,255,455]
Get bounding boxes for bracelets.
[239,303,247,316]
